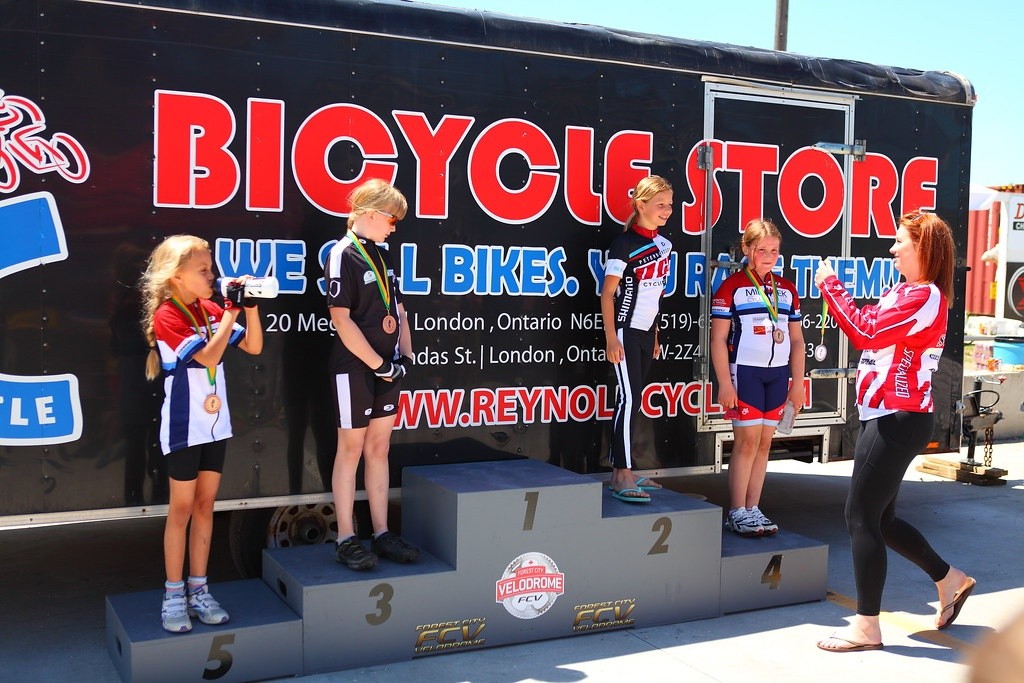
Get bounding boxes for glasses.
[378,210,398,227]
[911,211,926,222]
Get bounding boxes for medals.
[382,315,397,334]
[815,345,827,362]
[773,328,784,343]
[204,395,222,415]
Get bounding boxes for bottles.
[776,400,795,435]
[211,276,279,298]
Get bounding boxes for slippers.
[937,576,977,629]
[609,477,662,490]
[817,635,884,652]
[612,487,651,502]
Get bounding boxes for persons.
[599,173,673,502]
[110,300,172,505]
[711,221,805,538]
[141,235,263,632]
[522,277,615,474]
[813,210,976,652]
[322,177,421,569]
[281,294,336,495]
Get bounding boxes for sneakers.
[371,532,420,564]
[187,585,229,624]
[334,535,375,571]
[161,591,193,632]
[725,507,764,534]
[748,506,778,535]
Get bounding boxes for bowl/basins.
[993,336,1024,365]
